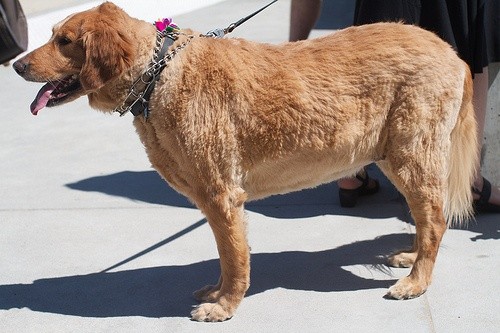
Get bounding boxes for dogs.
[12,2,482,321]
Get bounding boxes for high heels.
[471,176,500,214]
[338,169,379,208]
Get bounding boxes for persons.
[287,0,323,44]
[336,0,500,208]
